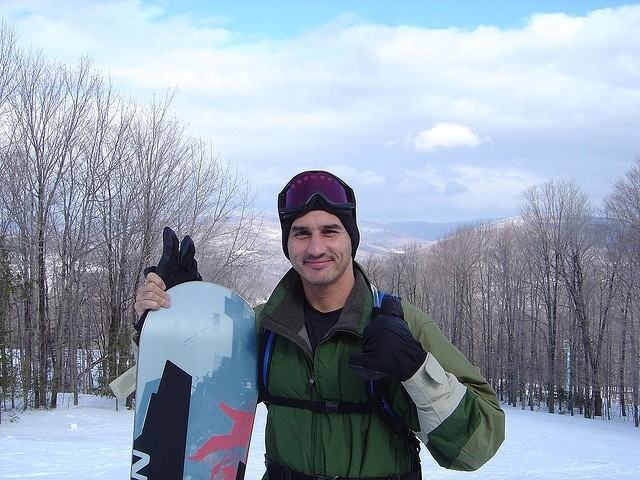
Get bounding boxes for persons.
[108,169,504,479]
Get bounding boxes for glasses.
[278,170,356,221]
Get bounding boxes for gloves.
[361,307,428,382]
[133,226,203,333]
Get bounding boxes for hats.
[282,202,359,261]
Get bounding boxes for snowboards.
[130,281,260,480]
